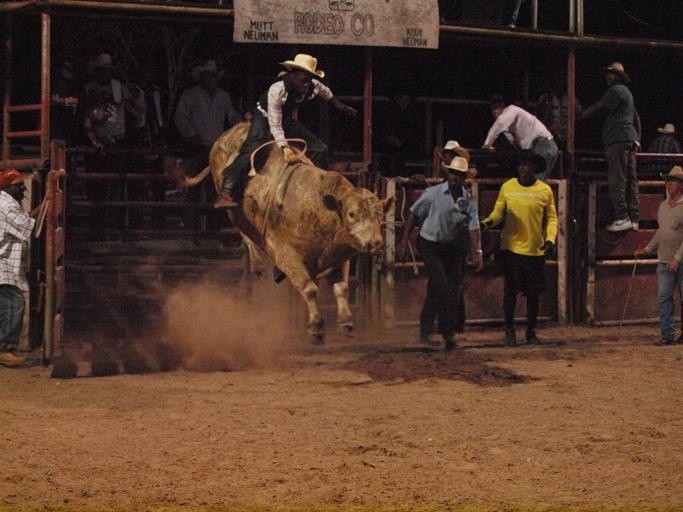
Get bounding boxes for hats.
[279,54,325,79]
[436,140,471,162]
[506,149,547,173]
[602,61,630,86]
[441,156,478,178]
[1,168,25,190]
[660,165,683,182]
[190,59,225,81]
[657,123,678,134]
[85,54,119,76]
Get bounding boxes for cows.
[168,118,395,349]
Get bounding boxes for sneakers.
[213,192,238,208]
[0,351,27,365]
[631,222,639,230]
[419,334,441,346]
[605,216,633,232]
[654,338,676,346]
[677,334,683,343]
[504,328,516,346]
[525,330,540,345]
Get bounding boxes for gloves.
[327,95,359,120]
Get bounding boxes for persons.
[633,167,682,346]
[0,168,46,367]
[438,135,471,335]
[479,150,559,346]
[400,154,485,355]
[59,54,683,235]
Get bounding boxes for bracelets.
[472,249,483,256]
